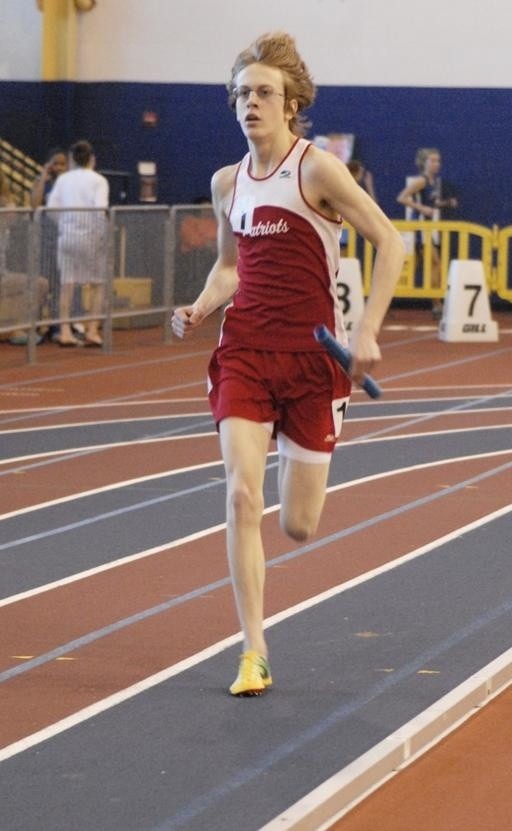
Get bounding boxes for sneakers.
[221,650,282,700]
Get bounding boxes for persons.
[45,140,110,346]
[171,32,405,697]
[347,159,374,200]
[31,148,68,342]
[178,198,218,252]
[397,146,457,320]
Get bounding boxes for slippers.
[2,320,107,350]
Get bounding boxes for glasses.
[229,84,288,103]
